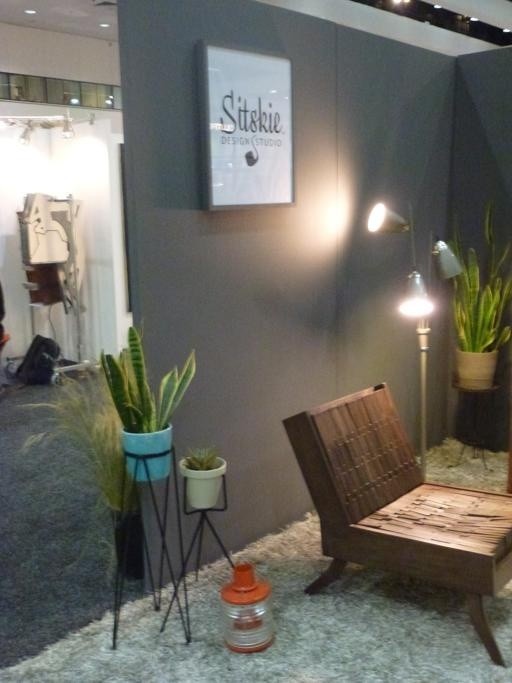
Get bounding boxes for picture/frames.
[196,39,295,212]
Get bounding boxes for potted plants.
[100,326,197,482]
[178,445,227,510]
[447,200,512,392]
[17,352,144,580]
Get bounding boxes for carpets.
[0,435,512,683]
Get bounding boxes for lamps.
[7,119,75,142]
[366,202,463,483]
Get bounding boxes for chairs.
[282,382,512,669]
